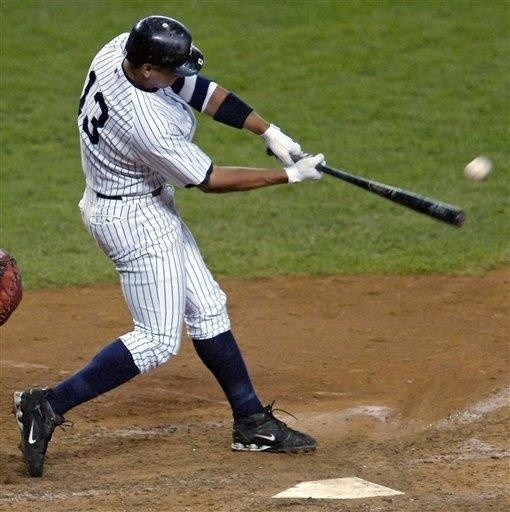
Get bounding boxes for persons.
[11,15,327,477]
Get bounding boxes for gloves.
[259,123,304,167]
[281,153,327,186]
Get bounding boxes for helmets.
[121,14,206,77]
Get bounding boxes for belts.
[96,186,163,203]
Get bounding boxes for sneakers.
[229,405,320,455]
[12,385,67,480]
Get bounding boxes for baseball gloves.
[0,251,24,326]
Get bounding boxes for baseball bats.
[265,146,464,226]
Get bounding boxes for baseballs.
[465,157,493,181]
